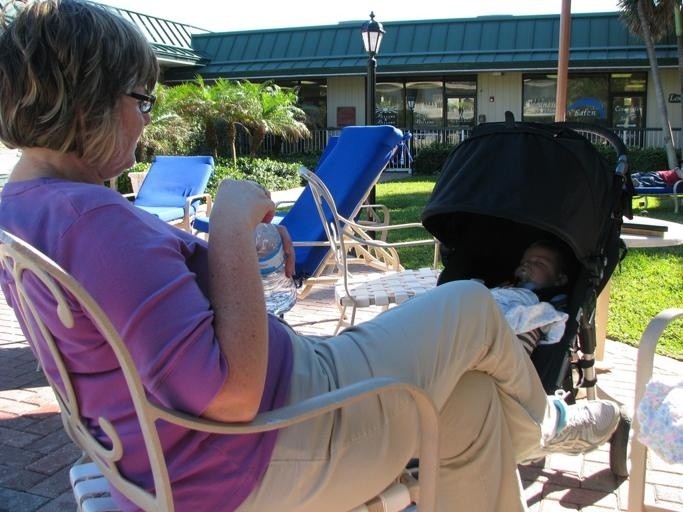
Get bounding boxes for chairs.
[299,168,443,335]
[0,227,440,512]
[128,155,212,237]
[282,127,401,298]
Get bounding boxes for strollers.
[404,117,640,482]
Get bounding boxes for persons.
[0,0,620,512]
[489,237,577,359]
[631,167,683,187]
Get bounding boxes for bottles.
[253,221,296,318]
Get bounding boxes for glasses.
[124,92,156,113]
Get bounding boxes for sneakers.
[519,389,620,467]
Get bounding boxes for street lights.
[359,9,389,257]
